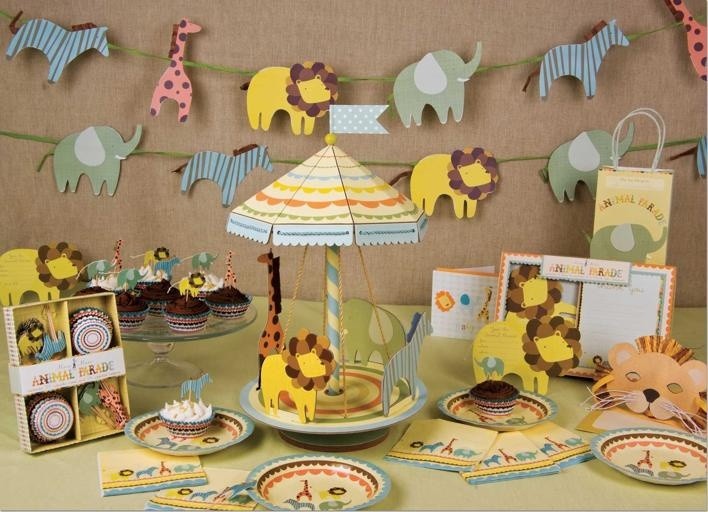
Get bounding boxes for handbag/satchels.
[589,107,675,267]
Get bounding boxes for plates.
[439,385,558,433]
[242,453,391,512]
[591,426,707,487]
[121,403,254,457]
